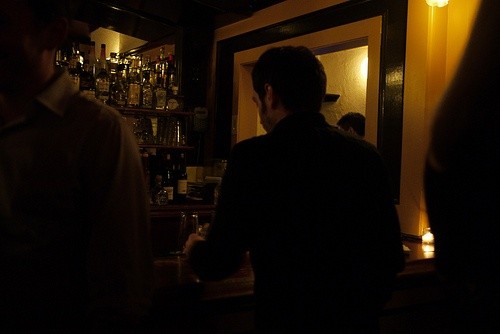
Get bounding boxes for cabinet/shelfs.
[110,103,227,255]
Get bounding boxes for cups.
[168,120,183,147]
[178,212,198,244]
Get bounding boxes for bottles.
[141,149,188,206]
[55,39,182,111]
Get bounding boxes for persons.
[425,0,500,334]
[338,113,377,152]
[183,46,405,334]
[0,0,154,334]
